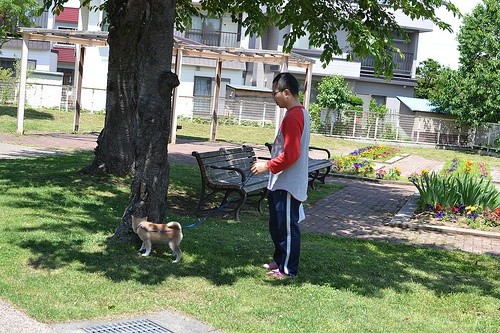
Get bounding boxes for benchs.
[191,146,272,221]
[265,142,333,190]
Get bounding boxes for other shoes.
[264,262,278,269]
[266,270,290,279]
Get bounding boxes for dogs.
[130,215,184,264]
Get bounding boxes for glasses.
[272,91,280,97]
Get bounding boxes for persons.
[249,72,310,280]
